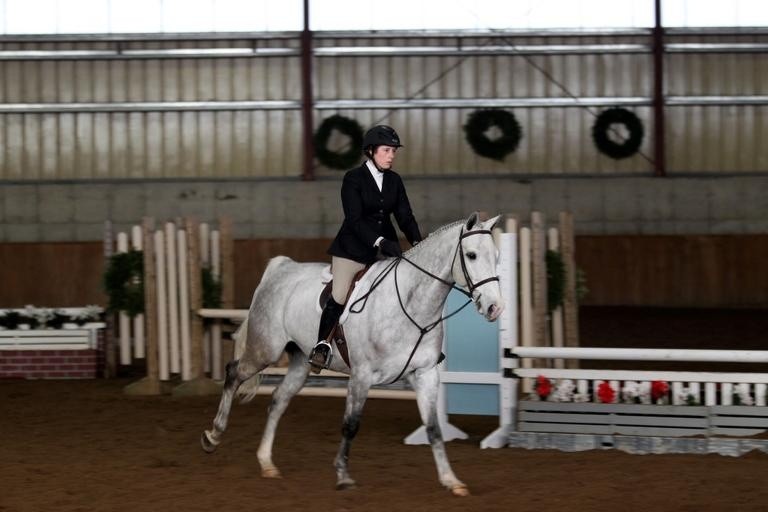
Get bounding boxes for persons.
[303,123,424,374]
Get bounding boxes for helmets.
[362,124,404,159]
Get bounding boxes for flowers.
[649,380,670,405]
[533,376,552,401]
[598,380,616,403]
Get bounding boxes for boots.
[311,293,346,374]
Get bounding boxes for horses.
[201,210,504,499]
